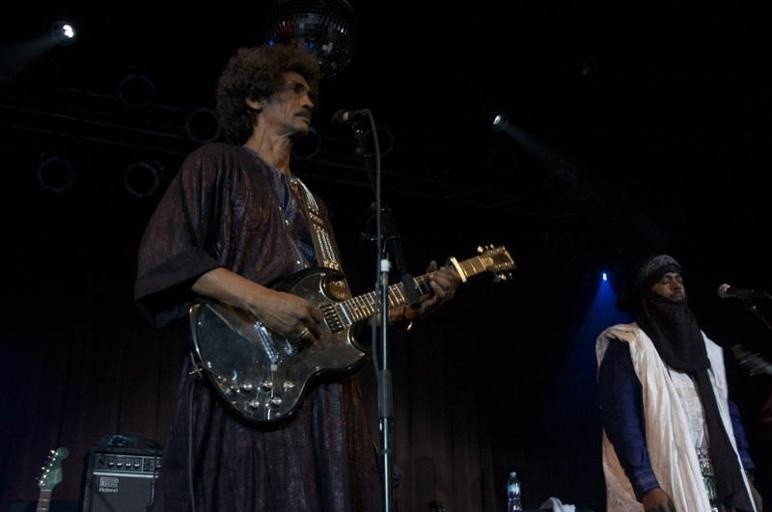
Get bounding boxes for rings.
[297,326,310,339]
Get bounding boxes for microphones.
[717,283,767,300]
[330,107,369,125]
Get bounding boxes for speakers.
[78,453,162,512]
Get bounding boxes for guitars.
[189,244,516,424]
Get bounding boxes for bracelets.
[399,302,408,321]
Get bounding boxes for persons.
[127,41,463,511]
[591,252,764,511]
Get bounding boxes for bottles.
[506,470,522,511]
